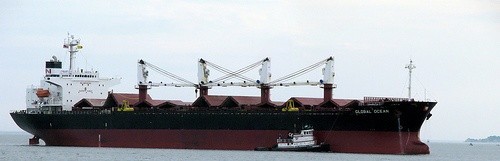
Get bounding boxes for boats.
[253,121,331,153]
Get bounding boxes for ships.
[8,31,438,156]
